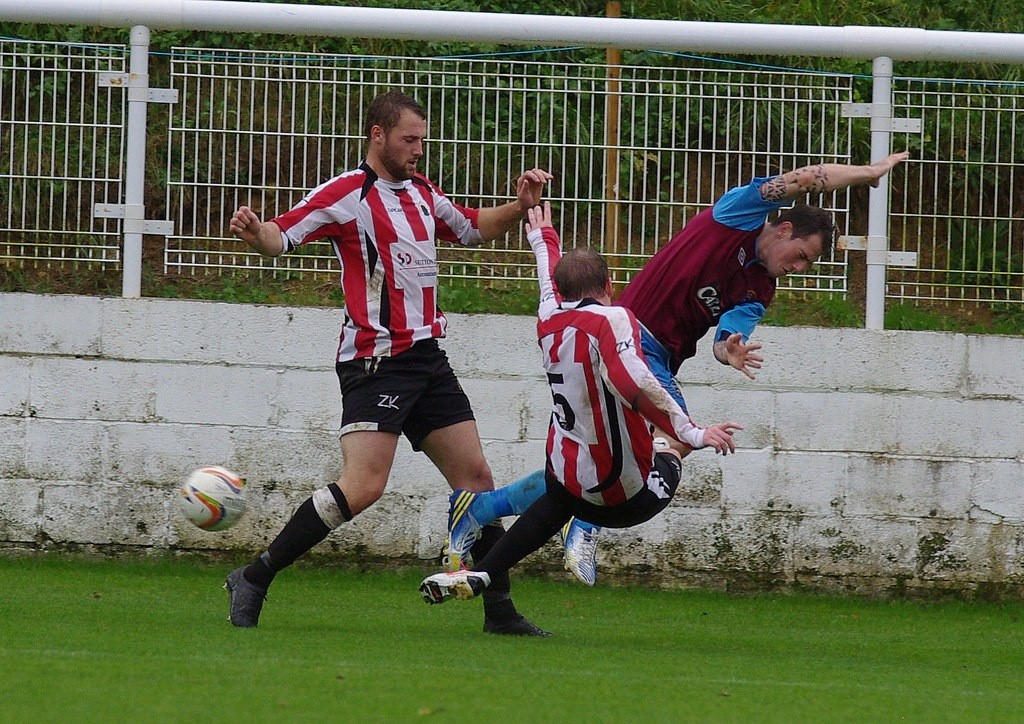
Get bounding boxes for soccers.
[180,466,248,532]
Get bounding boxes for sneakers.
[419,569,491,604]
[560,515,601,586]
[223,563,267,629]
[440,489,486,573]
[482,615,554,638]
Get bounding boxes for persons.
[225,87,558,639]
[416,200,745,606]
[442,150,910,572]
